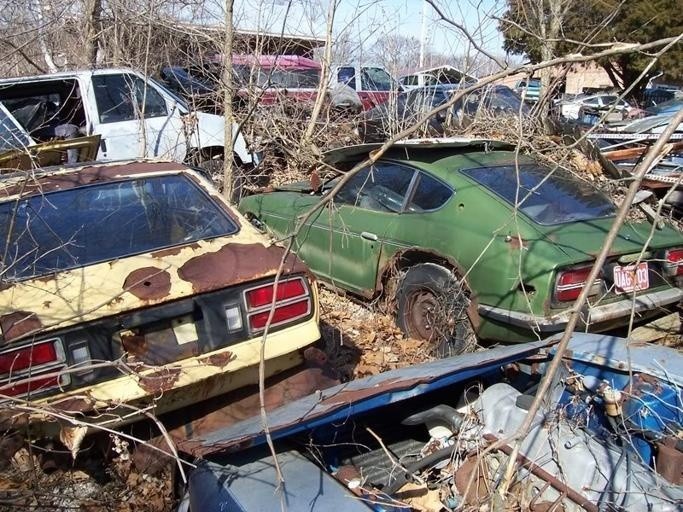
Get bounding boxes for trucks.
[208,55,406,113]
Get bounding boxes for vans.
[0,67,253,204]
